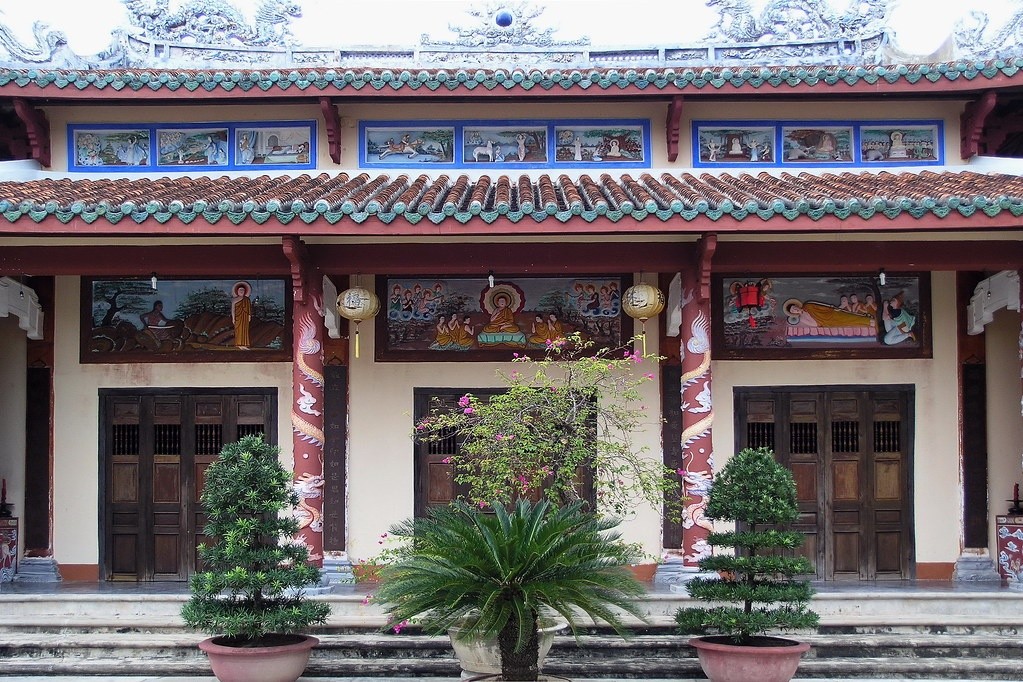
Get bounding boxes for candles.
[2,479,6,496]
[1013,482,1019,500]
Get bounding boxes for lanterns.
[336,274,381,359]
[622,273,665,359]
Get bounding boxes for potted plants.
[670,447,822,682]
[367,490,645,682]
[178,432,334,682]
